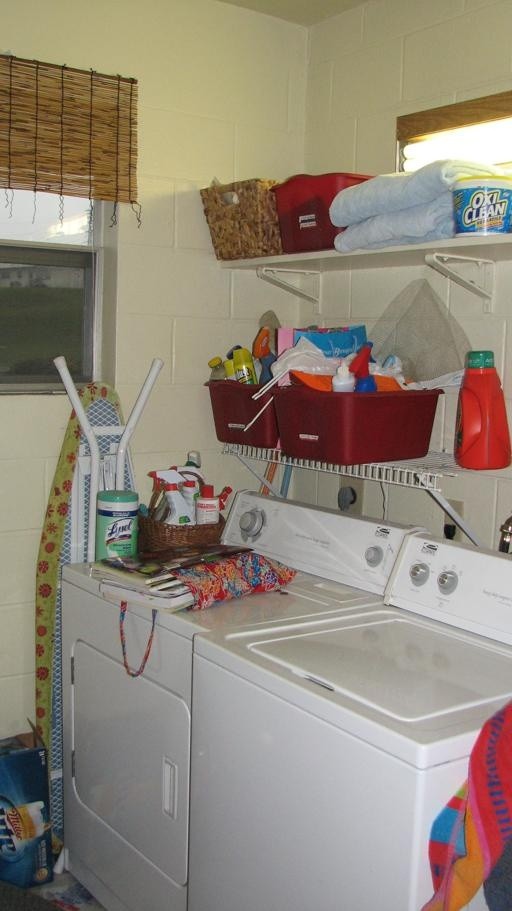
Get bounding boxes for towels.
[325,159,508,229]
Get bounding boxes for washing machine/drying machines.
[60,501,405,910]
[189,531,512,910]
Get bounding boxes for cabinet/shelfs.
[203,232,497,547]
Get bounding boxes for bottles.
[208,346,256,386]
[182,480,219,524]
[333,360,355,394]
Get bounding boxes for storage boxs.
[205,376,278,449]
[272,383,445,464]
[268,173,374,254]
[202,175,286,262]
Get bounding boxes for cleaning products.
[147,451,232,525]
[223,326,278,384]
[332,342,378,392]
[454,351,511,470]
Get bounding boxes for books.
[89,551,196,615]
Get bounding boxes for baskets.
[138,470,225,553]
